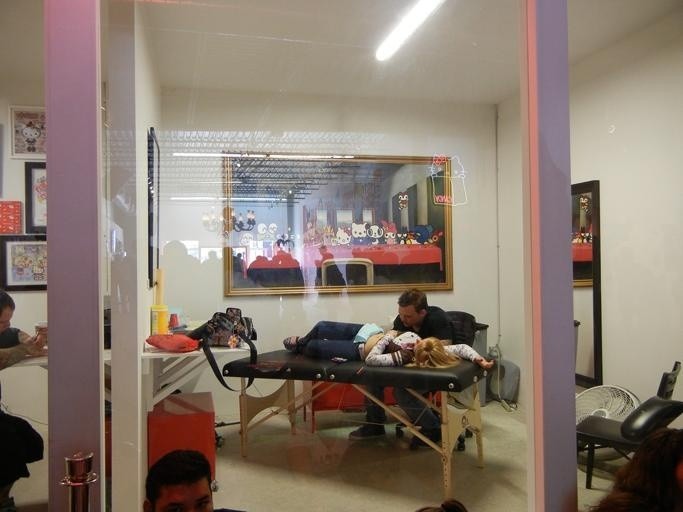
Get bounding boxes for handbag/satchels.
[188,307,258,392]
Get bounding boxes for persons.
[143,450,246,512]
[0,290,48,356]
[350,288,454,448]
[282,320,494,369]
[591,427,683,512]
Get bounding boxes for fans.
[574,386,643,466]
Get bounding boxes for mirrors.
[572,181,601,391]
[219,151,455,298]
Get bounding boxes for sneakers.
[349,424,386,439]
[414,428,441,448]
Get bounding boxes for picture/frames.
[1,234,46,292]
[8,105,48,159]
[25,161,48,234]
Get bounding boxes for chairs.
[394,311,477,449]
[575,361,681,490]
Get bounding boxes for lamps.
[231,207,257,232]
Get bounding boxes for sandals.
[283,336,300,345]
[285,345,297,351]
